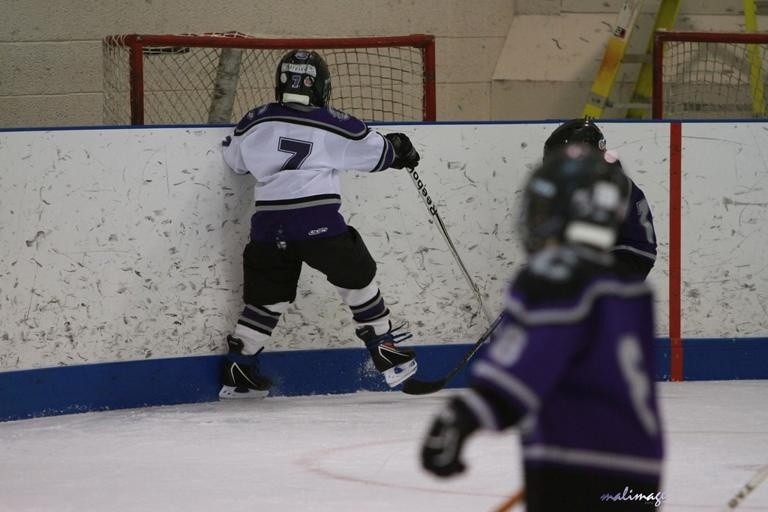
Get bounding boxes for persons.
[420,121,664,511]
[220,49,421,390]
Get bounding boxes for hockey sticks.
[402,307,507,395]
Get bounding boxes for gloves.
[386,133,420,168]
[421,396,479,477]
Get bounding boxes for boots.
[222,335,271,390]
[356,325,416,373]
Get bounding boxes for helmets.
[275,50,332,108]
[519,119,630,251]
